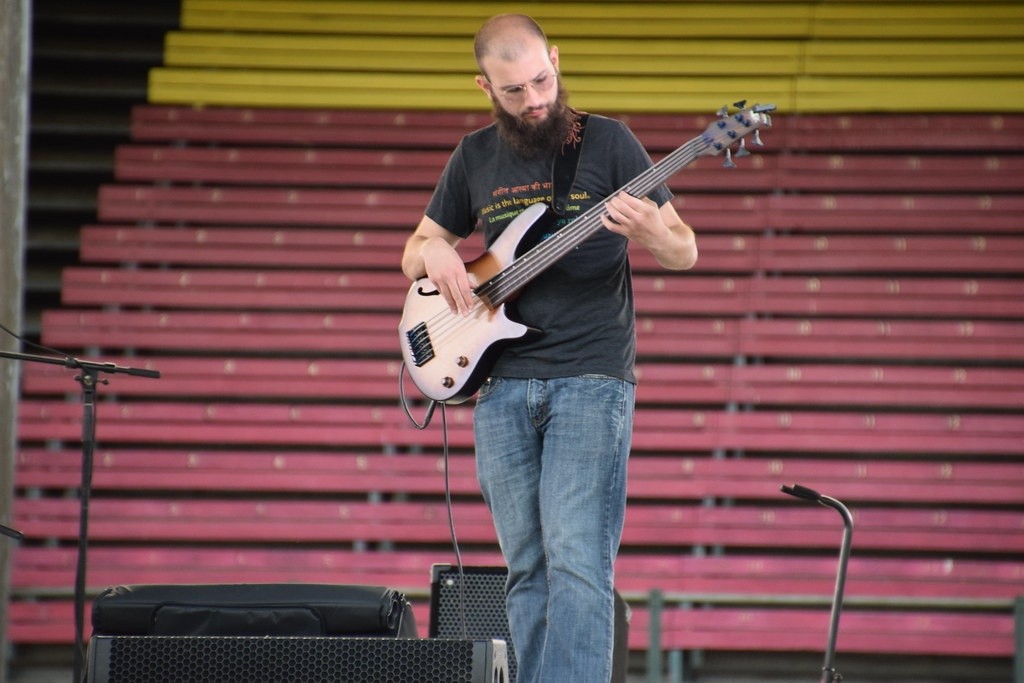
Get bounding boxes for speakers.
[429,563,630,683]
[91,584,419,638]
[85,636,509,683]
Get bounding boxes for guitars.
[394,97,777,405]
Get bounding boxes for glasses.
[487,65,561,103]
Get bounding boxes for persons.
[399,11,699,681]
